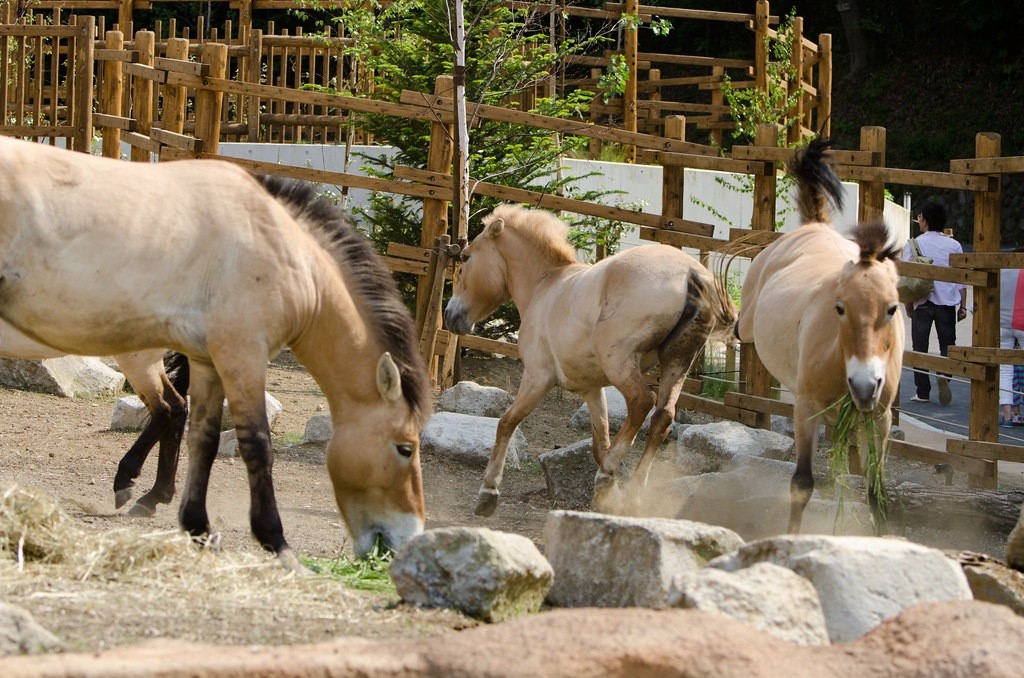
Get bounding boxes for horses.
[442,202,772,525]
[733,106,905,534]
[0,132,433,581]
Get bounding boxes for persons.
[900,196,1024,428]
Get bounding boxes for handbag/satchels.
[896,237,933,304]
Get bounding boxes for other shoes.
[936,371,952,406]
[998,417,1013,428]
[1013,416,1024,426]
[910,394,929,403]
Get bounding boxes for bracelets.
[960,306,967,311]
